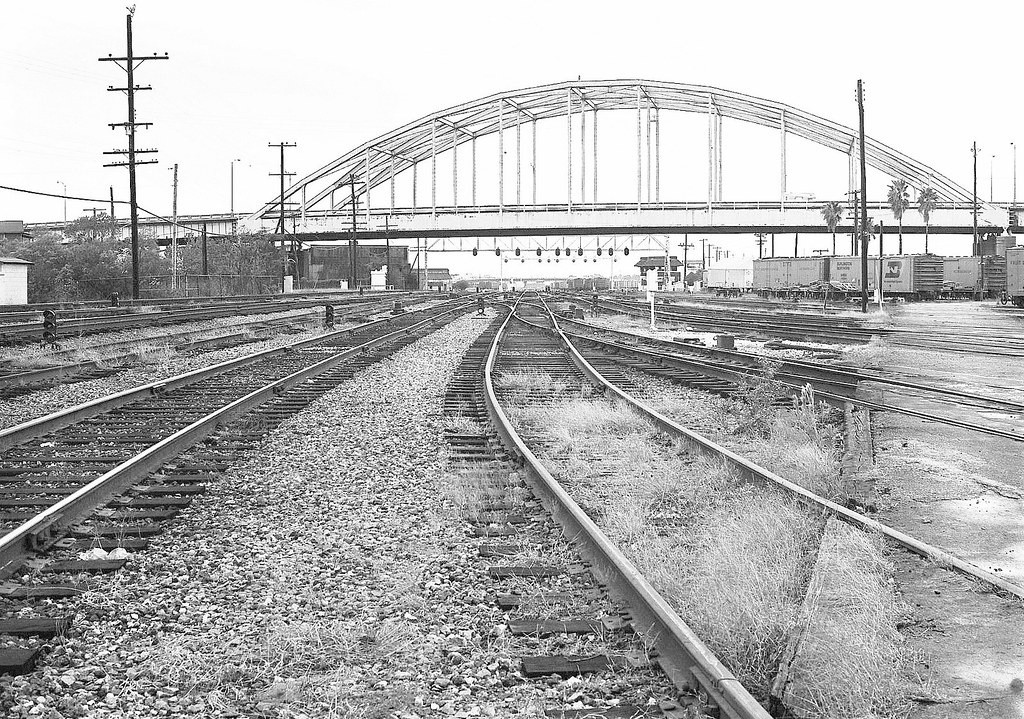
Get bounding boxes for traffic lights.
[326,305,333,327]
[43,308,56,344]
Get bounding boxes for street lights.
[57,180,67,226]
[990,155,996,201]
[854,78,871,313]
[971,140,982,256]
[231,158,241,215]
[1010,142,1017,208]
